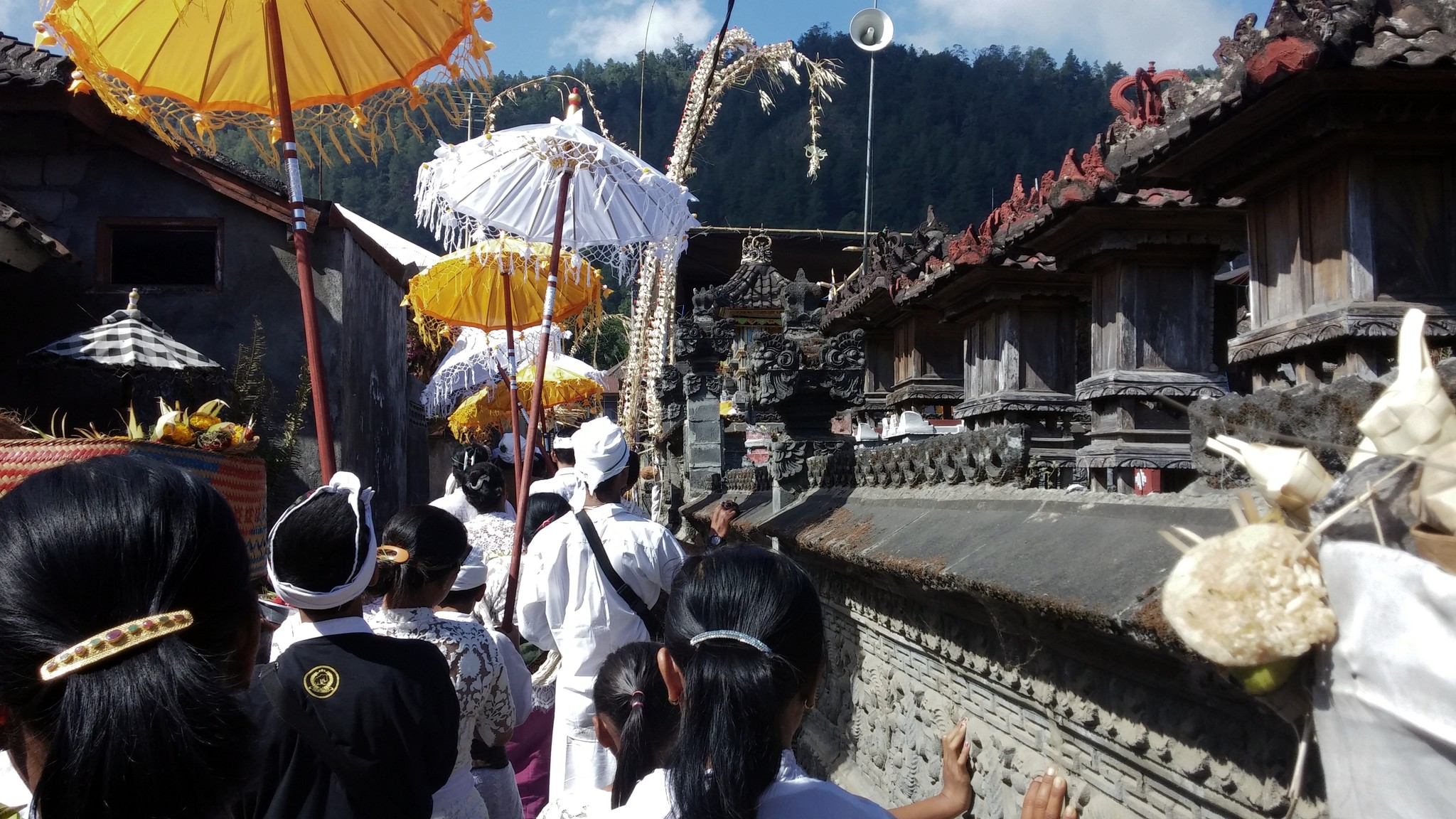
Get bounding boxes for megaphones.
[849,8,892,53]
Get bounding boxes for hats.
[597,451,640,493]
[498,433,526,464]
[568,417,631,515]
[450,547,489,592]
[553,430,573,450]
[266,472,378,609]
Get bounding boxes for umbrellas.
[416,89,703,634]
[32,0,494,488]
[448,350,606,442]
[407,231,606,509]
[421,324,561,478]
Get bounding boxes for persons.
[0,414,1076,819]
